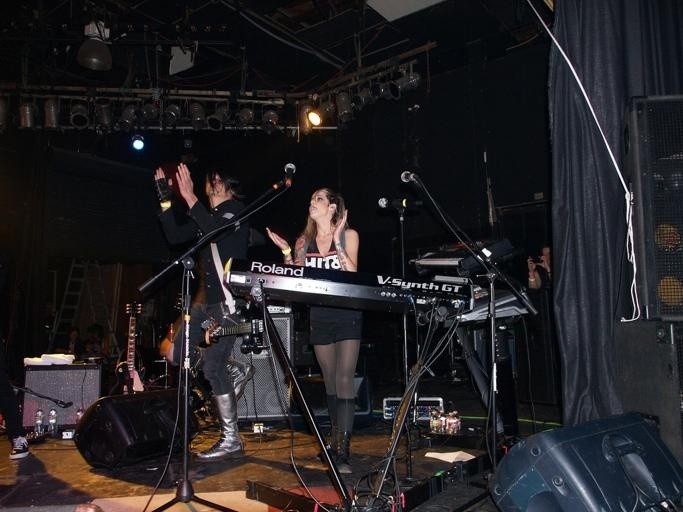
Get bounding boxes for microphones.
[399,170,419,184]
[375,197,424,209]
[282,162,297,190]
[249,285,266,304]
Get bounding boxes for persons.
[61,326,80,353]
[151,162,248,461]
[526,245,552,290]
[0,365,30,461]
[264,186,364,466]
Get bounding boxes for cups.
[61,432,72,440]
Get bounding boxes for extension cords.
[61,428,76,440]
[253,422,264,435]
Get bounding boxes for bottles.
[47,407,59,439]
[34,407,44,436]
[428,408,460,432]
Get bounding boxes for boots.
[193,347,254,401]
[191,392,245,463]
[335,431,352,474]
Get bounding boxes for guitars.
[202,300,265,354]
[110,298,143,396]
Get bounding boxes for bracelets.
[282,246,292,255]
[528,278,536,282]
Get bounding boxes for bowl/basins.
[80,357,103,363]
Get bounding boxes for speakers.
[608,319,682,467]
[21,361,104,432]
[621,94,683,319]
[73,388,204,469]
[232,303,297,425]
[487,409,683,512]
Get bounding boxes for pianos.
[222,257,475,312]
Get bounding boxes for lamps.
[0,50,421,136]
[75,0,114,74]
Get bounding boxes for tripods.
[149,341,237,512]
[453,309,501,512]
